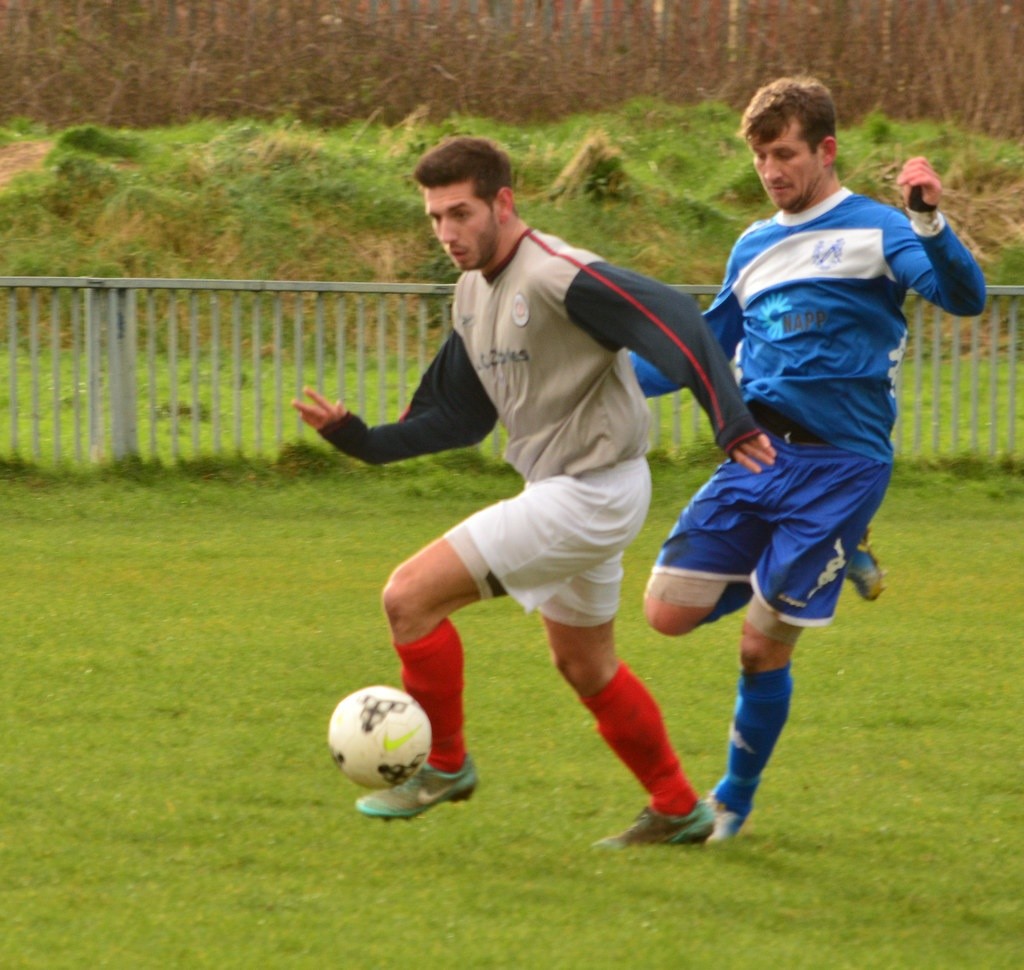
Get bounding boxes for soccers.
[327,684,434,791]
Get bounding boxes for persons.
[291,137,776,846]
[623,77,987,846]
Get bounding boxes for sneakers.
[590,799,717,850]
[355,753,475,819]
[844,526,881,600]
[706,791,746,843]
